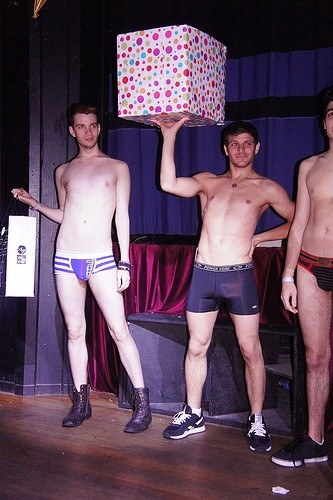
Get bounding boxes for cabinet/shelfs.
[119,312,305,439]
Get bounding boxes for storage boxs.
[117,24,227,127]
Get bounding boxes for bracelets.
[118,268,130,270]
[118,261,131,267]
[283,277,293,283]
[32,203,41,210]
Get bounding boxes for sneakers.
[271,432,328,467]
[247,413,272,452]
[162,405,206,439]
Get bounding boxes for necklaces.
[231,179,237,187]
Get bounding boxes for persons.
[271,89,333,468]
[10,103,152,432]
[151,118,295,450]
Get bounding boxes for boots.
[61,384,92,427]
[124,387,152,433]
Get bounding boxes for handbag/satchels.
[0,193,37,297]
